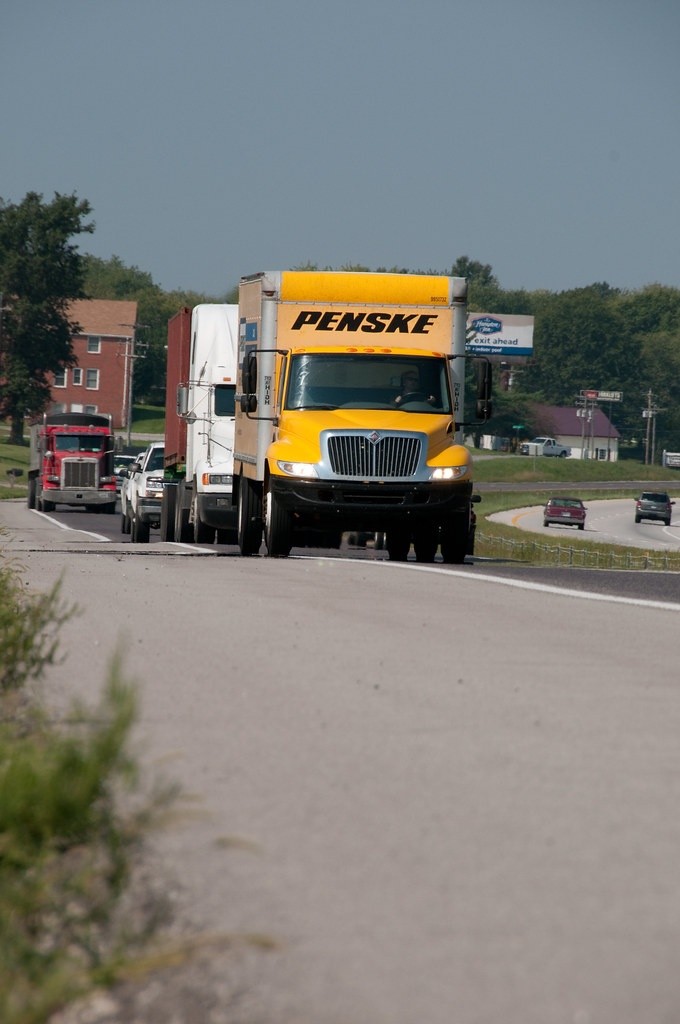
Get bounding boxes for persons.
[391,370,436,404]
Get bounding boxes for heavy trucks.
[158,302,239,546]
[26,410,122,516]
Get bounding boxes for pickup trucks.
[517,437,572,458]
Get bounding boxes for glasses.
[406,377,418,382]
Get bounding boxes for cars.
[541,496,588,530]
[113,441,166,544]
[634,490,676,526]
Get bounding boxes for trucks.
[229,269,493,566]
